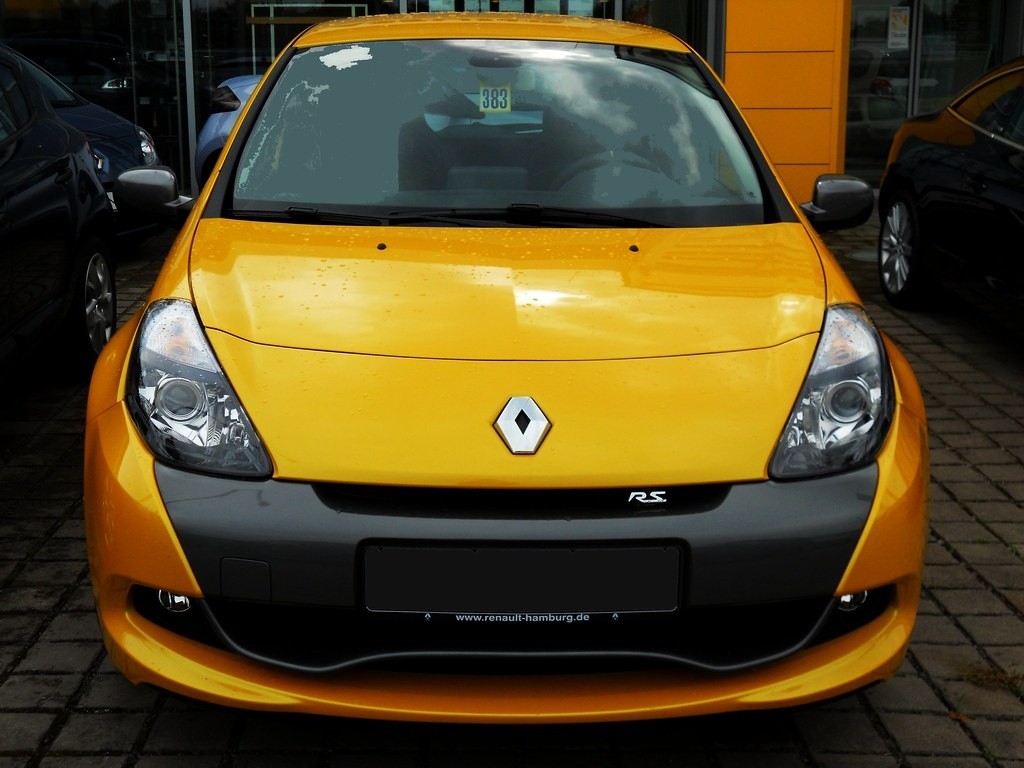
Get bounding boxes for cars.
[12,51,162,243]
[85,9,929,726]
[873,62,1024,328]
[0,50,124,398]
